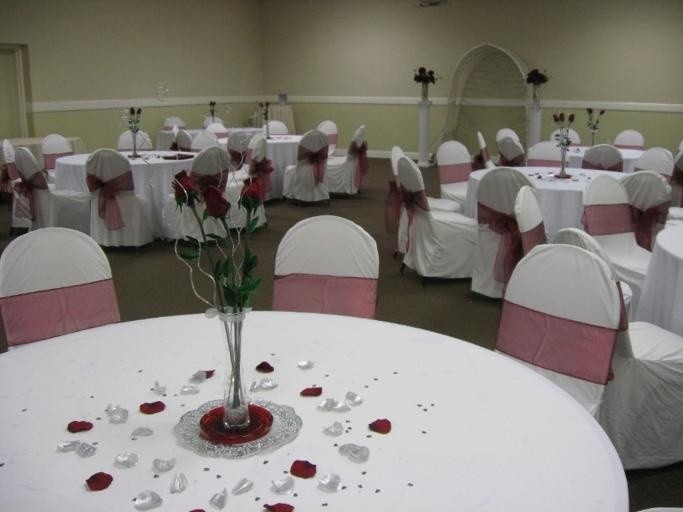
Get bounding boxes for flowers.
[552,113,575,145]
[258,101,270,120]
[124,106,142,132]
[585,107,605,129]
[526,68,548,85]
[208,98,216,115]
[412,65,443,86]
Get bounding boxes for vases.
[532,85,540,103]
[421,83,428,100]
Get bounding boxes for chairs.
[495,243,620,417]
[273,214,380,320]
[552,227,681,467]
[2,114,681,291]
[2,227,122,349]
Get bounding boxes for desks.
[55,151,195,196]
[467,166,632,229]
